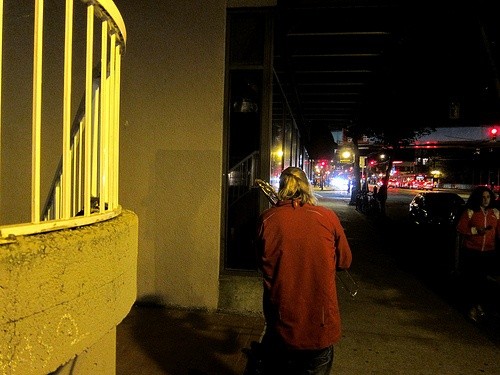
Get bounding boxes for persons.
[378,177,388,214]
[256,166,353,375]
[456,186,500,322]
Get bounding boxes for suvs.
[388,179,397,188]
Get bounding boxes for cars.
[409,190,466,233]
[397,180,433,190]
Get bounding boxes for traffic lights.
[321,161,325,166]
[490,128,498,142]
[339,148,352,163]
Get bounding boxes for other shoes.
[468,304,484,321]
